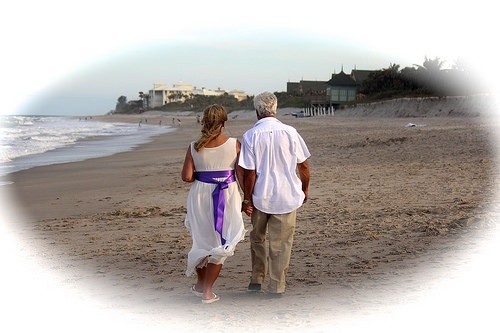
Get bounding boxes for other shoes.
[248,283,261,290]
[271,292,282,298]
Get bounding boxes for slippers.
[190,284,203,297]
[202,291,220,304]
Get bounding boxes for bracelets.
[243,199,250,204]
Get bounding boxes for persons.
[172,118,181,127]
[181,103,253,304]
[237,90,312,298]
[196,114,201,123]
